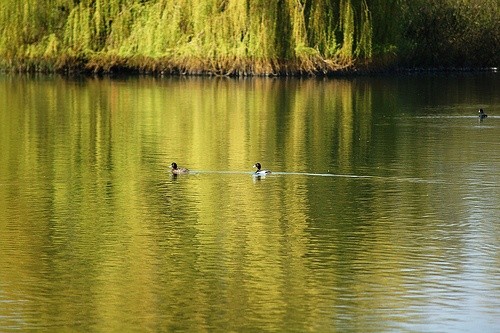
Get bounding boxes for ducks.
[478,109,487,118]
[169,162,190,174]
[253,163,271,173]
